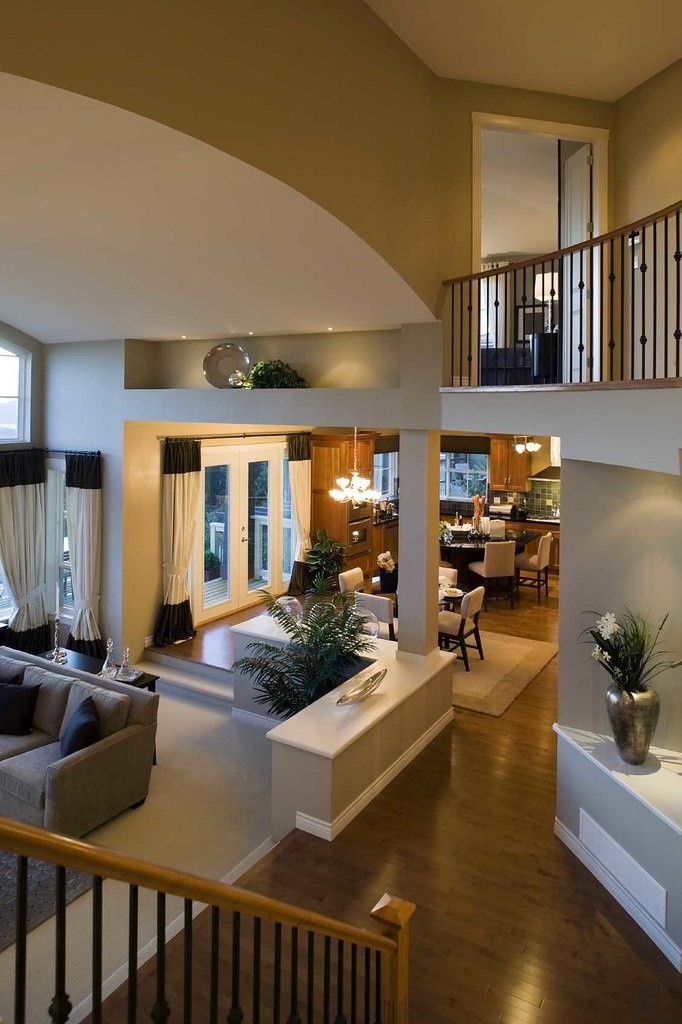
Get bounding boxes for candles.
[55,581,59,618]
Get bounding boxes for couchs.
[0,645,159,840]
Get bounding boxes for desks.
[439,528,541,599]
[480,348,532,387]
[366,580,468,651]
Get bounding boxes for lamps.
[328,427,382,510]
[534,271,560,332]
[512,434,542,454]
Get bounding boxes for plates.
[203,343,249,388]
[444,588,463,597]
[335,669,387,705]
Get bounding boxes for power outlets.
[494,497,500,504]
[545,499,553,506]
[508,497,513,503]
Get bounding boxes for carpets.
[440,630,559,720]
[0,848,106,956]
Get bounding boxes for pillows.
[0,683,46,736]
[61,695,100,758]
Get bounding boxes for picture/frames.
[517,305,550,343]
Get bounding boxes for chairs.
[354,591,399,641]
[340,567,365,606]
[439,567,458,649]
[468,541,516,613]
[515,532,553,605]
[438,586,485,672]
[531,332,560,386]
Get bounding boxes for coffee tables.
[37,646,160,766]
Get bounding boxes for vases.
[604,682,663,766]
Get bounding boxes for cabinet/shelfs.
[308,433,382,580]
[488,434,534,491]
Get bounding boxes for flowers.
[577,602,682,700]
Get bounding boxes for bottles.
[454,511,462,527]
[229,369,245,388]
[102,638,117,678]
[117,648,134,680]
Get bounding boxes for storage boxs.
[379,563,399,592]
[490,528,505,537]
[490,519,505,528]
